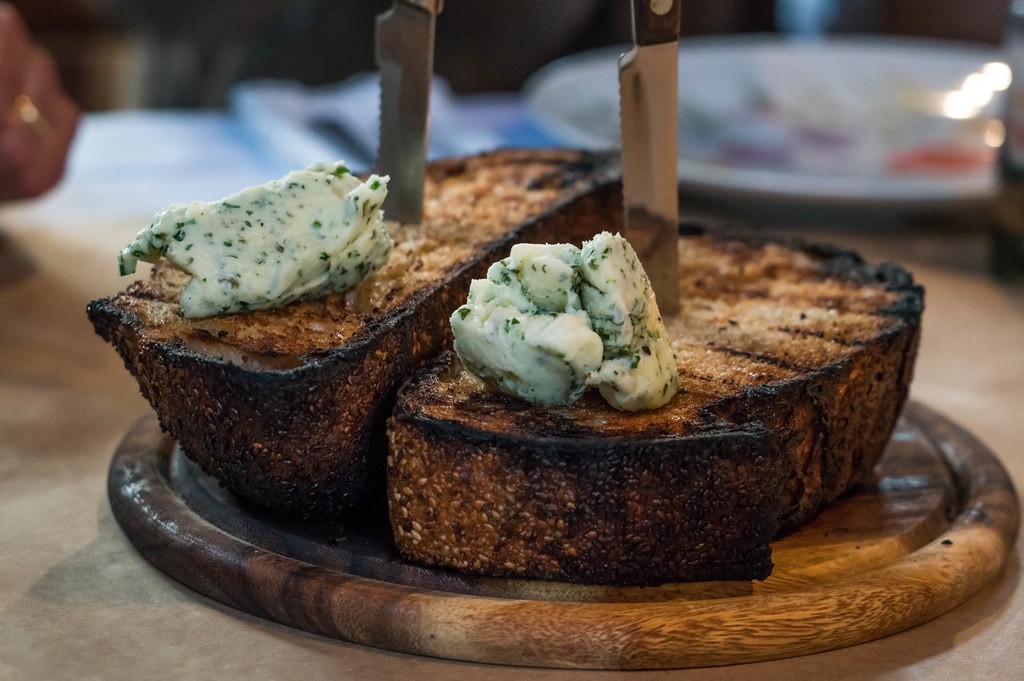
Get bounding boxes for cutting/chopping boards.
[108,398,1021,671]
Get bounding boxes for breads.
[388,230,923,579]
[84,143,620,534]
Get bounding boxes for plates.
[522,43,1004,215]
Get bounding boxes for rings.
[14,96,40,126]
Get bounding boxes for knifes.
[373,0,444,226]
[614,0,681,318]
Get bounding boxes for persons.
[0,4,81,204]
